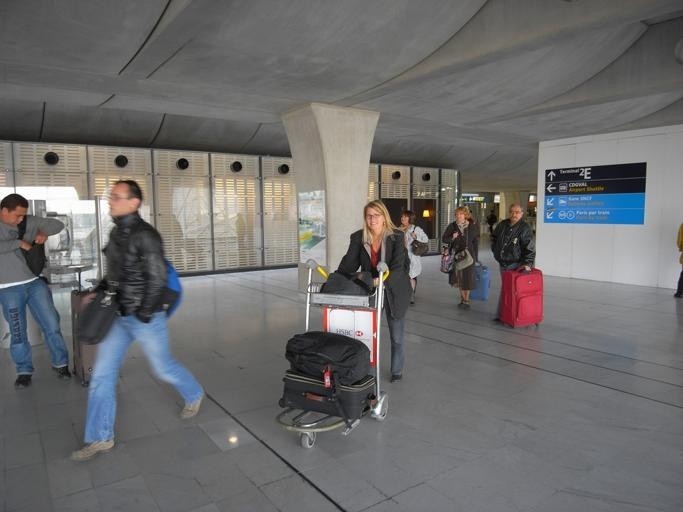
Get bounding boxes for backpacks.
[285,330,371,387]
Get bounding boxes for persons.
[674,222,683,297]
[69,179,206,461]
[0,193,74,390]
[491,201,536,322]
[442,206,497,310]
[397,210,429,306]
[337,199,411,382]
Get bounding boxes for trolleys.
[273,258,390,450]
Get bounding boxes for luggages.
[469,262,491,301]
[71,268,100,387]
[279,368,376,419]
[499,266,543,328]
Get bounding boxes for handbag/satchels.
[78,291,120,345]
[439,246,454,273]
[411,240,429,256]
[321,270,374,296]
[452,250,474,272]
[164,259,183,318]
[25,240,48,277]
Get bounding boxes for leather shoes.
[391,375,402,384]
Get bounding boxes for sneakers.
[179,393,203,421]
[52,366,72,381]
[71,438,114,461]
[15,375,32,388]
